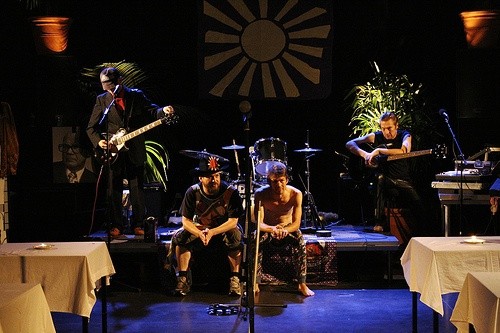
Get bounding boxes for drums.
[253,137,289,174]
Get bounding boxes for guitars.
[94,109,179,163]
[350,141,433,178]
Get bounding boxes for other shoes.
[373,219,388,231]
[134,228,144,235]
[119,234,126,238]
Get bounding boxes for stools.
[369,189,390,234]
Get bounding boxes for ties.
[68,172,76,183]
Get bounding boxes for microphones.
[440,109,451,119]
[113,85,122,95]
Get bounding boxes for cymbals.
[180,149,229,162]
[222,146,245,149]
[294,148,323,152]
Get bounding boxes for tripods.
[299,153,325,230]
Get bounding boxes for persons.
[253,165,315,295]
[346,113,431,233]
[171,156,243,295]
[53,133,92,183]
[87,67,174,236]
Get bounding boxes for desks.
[400,233,500,333]
[449,271,500,333]
[0,241,116,333]
[0,281,57,333]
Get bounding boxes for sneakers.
[175,279,190,293]
[228,276,241,296]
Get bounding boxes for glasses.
[58,144,80,153]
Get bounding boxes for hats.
[192,156,224,176]
[99,67,120,90]
